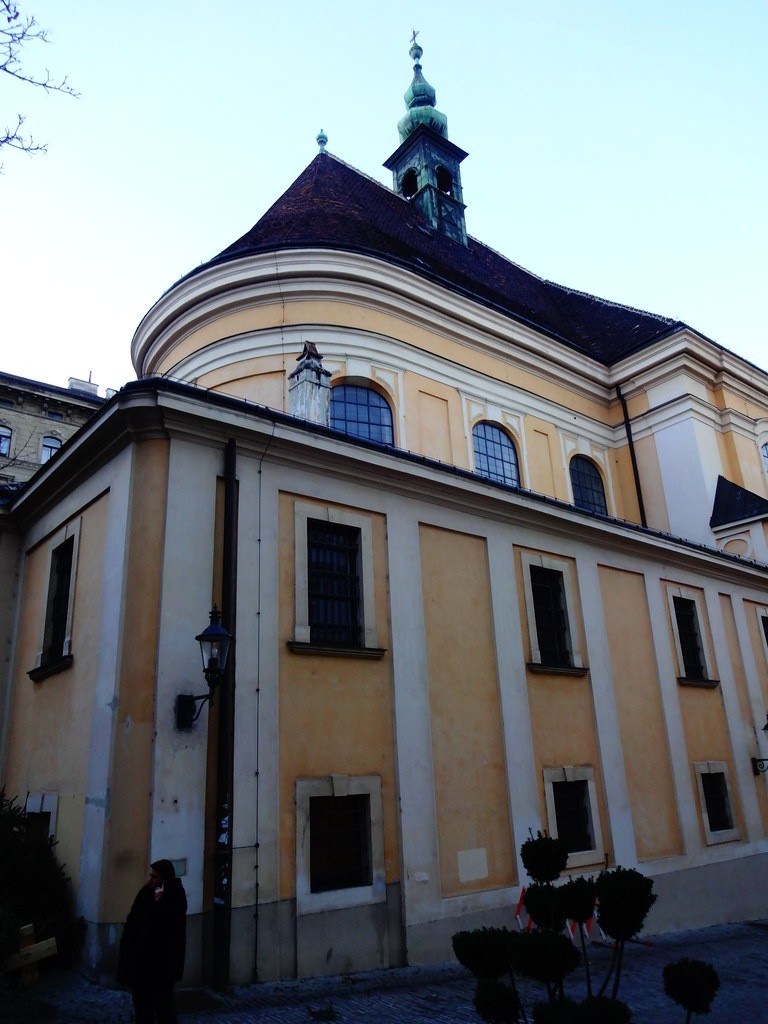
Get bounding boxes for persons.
[116,859,188,1024]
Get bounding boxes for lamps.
[173,606,230,731]
[751,714,768,777]
